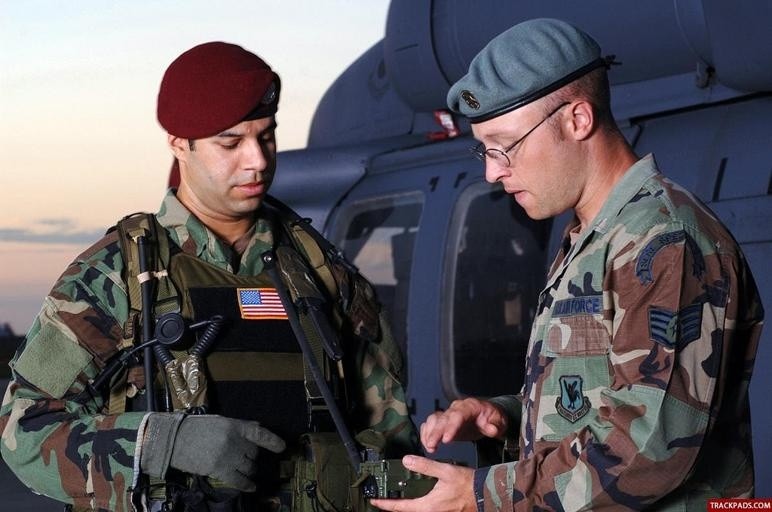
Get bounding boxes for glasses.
[463,101,572,167]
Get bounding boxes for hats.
[445,17,610,126]
[156,40,283,140]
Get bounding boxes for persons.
[1,42,422,512]
[369,18,765,512]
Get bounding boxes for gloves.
[139,411,287,494]
